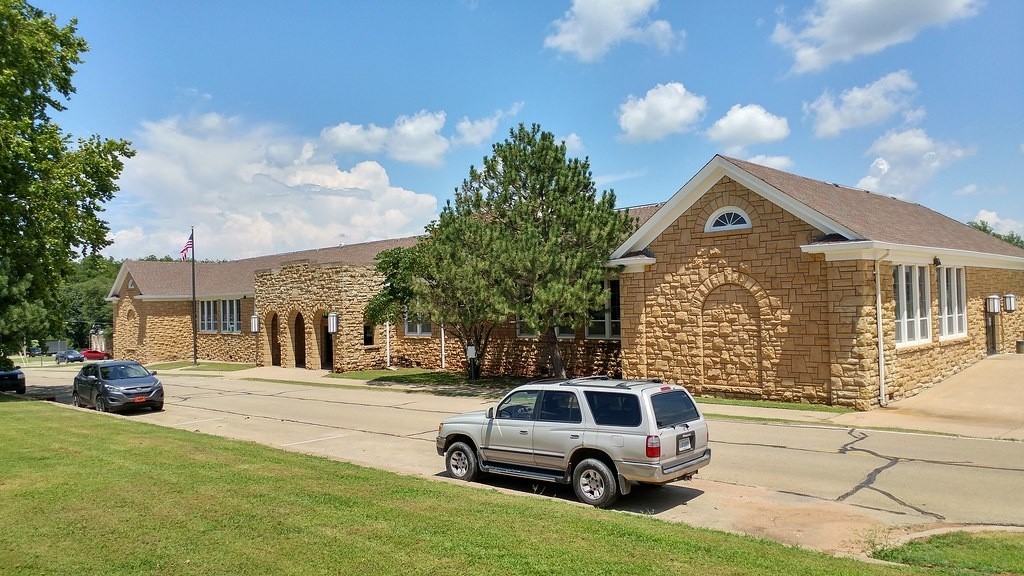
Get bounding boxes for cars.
[0,365,26,394]
[55,350,84,364]
[73,360,164,413]
[81,350,111,360]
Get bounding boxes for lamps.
[986,295,1000,314]
[328,313,338,333]
[251,315,259,333]
[1003,294,1016,312]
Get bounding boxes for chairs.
[532,392,556,419]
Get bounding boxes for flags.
[180,234,193,261]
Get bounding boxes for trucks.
[30,340,67,357]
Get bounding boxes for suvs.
[435,375,712,511]
